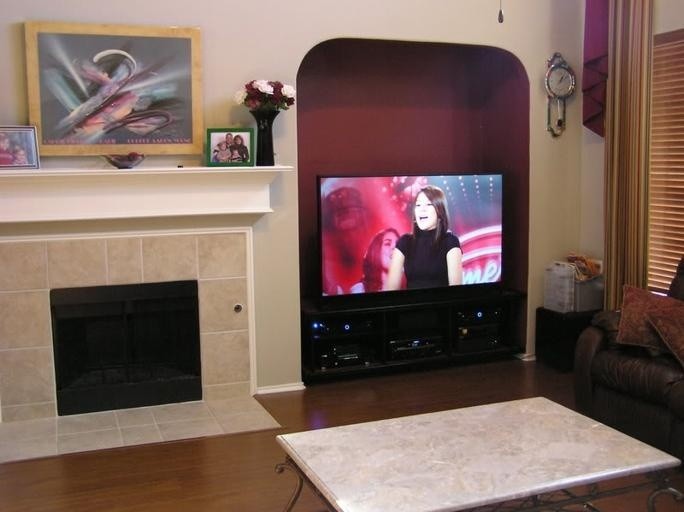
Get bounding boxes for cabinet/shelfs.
[301,284,524,386]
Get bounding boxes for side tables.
[536,306,605,374]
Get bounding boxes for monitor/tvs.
[315,173,510,311]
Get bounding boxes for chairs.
[573,254,684,459]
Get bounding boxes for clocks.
[543,52,576,137]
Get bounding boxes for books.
[549,252,603,283]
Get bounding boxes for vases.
[249,109,280,166]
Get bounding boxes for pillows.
[643,304,684,369]
[615,284,684,352]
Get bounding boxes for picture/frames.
[24,19,204,157]
[0,125,40,170]
[206,127,255,167]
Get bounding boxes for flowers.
[231,79,296,112]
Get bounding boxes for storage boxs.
[573,263,605,313]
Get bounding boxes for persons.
[349,227,400,294]
[0,131,28,165]
[386,186,465,291]
[212,133,249,162]
